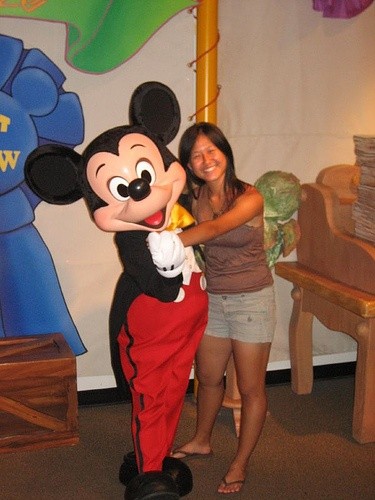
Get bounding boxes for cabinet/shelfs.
[271,162,375,445]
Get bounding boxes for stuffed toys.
[24,77,209,499]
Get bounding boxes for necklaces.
[206,183,230,220]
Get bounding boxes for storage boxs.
[0,331,80,453]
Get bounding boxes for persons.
[145,122,277,493]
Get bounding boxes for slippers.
[215,475,246,497]
[173,445,213,461]
